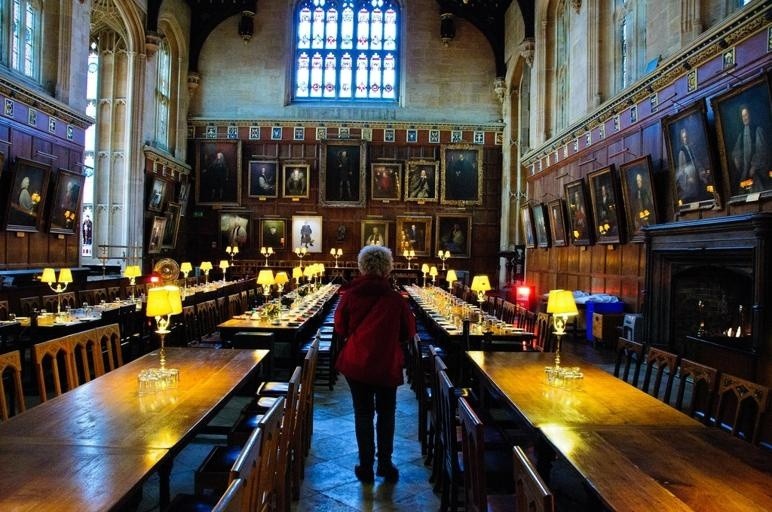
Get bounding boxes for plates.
[151,257,179,282]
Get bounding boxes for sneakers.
[355,465,374,483]
[376,463,398,477]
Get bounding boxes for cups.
[543,365,584,391]
[410,282,512,335]
[178,279,245,301]
[7,292,145,324]
[136,367,180,396]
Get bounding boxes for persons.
[333,244,415,482]
[523,102,771,247]
[18,175,92,244]
